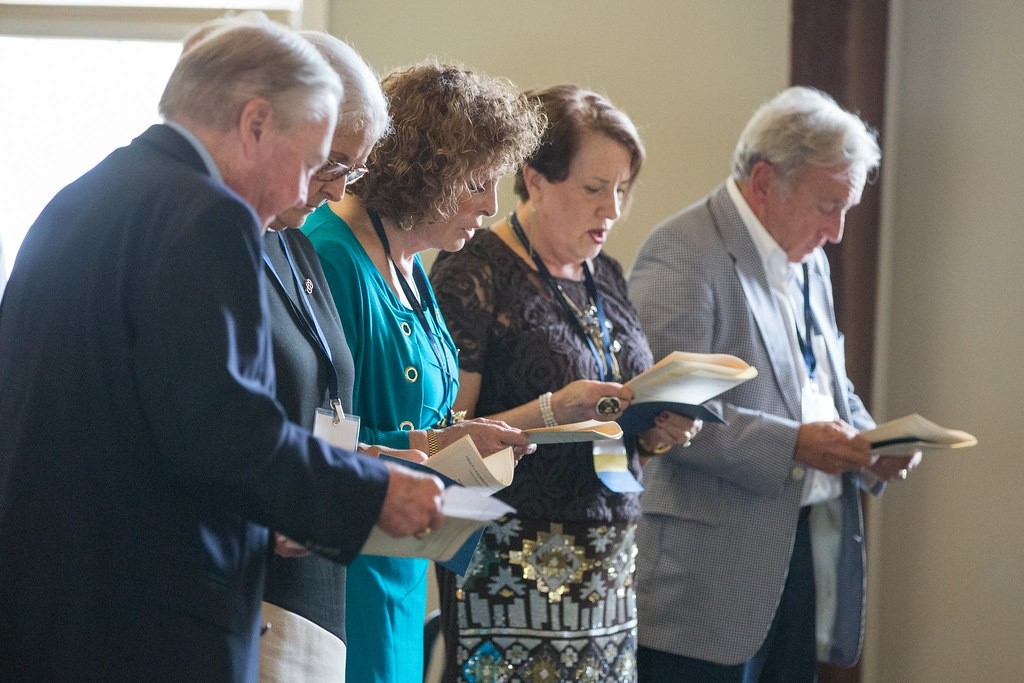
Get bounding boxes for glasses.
[317,158,368,186]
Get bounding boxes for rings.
[682,440,692,448]
[417,527,432,539]
[595,396,623,418]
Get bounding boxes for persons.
[0,18,447,683]
[626,86,923,683]
[427,84,704,683]
[296,65,513,683]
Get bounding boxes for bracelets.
[426,429,439,457]
[638,439,673,455]
[538,391,559,428]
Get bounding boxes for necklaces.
[506,213,621,382]
